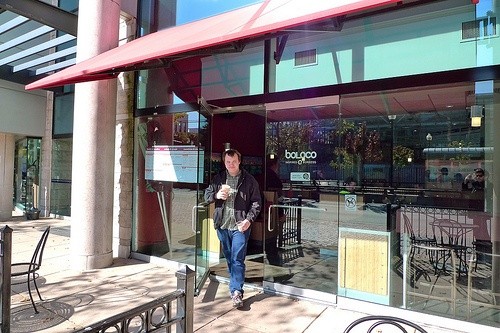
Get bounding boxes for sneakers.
[230,290,244,307]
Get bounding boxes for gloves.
[465,173,471,184]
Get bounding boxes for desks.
[429,222,481,277]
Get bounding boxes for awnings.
[25,0,400,92]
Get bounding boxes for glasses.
[476,174,483,177]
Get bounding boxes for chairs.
[432,219,478,280]
[10,225,51,314]
[401,213,437,264]
[342,316,428,333]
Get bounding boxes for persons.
[434,166,452,183]
[465,167,495,262]
[339,178,357,194]
[264,153,295,283]
[204,149,262,308]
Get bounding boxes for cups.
[222,184,229,196]
[236,221,243,232]
